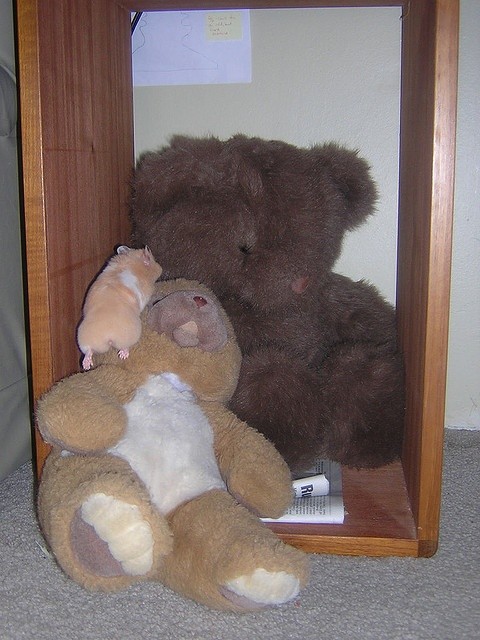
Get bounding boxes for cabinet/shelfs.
[13,0,460,559]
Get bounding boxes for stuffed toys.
[32,277,310,614]
[125,130,406,473]
[75,245,163,372]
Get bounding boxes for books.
[258,456,344,525]
